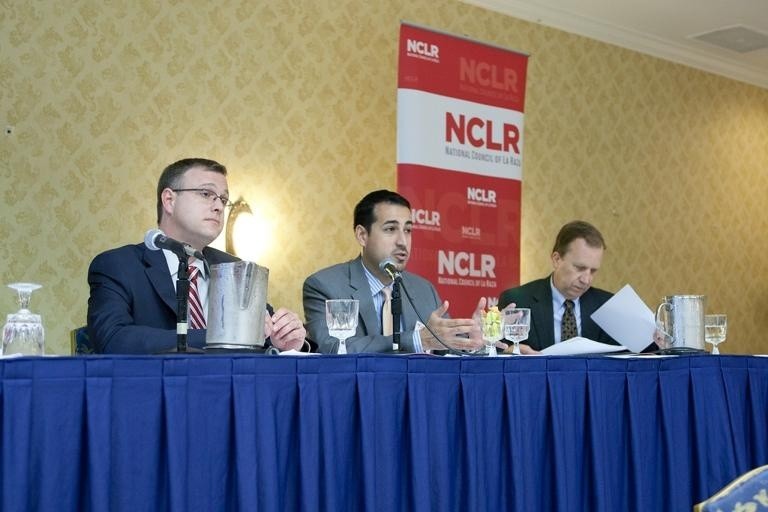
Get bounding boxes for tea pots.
[654,291,710,358]
[201,259,270,355]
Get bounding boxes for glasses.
[172,186,233,210]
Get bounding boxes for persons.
[85,158,320,356]
[497,220,670,354]
[303,189,525,354]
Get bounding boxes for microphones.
[379,258,402,282]
[144,229,204,261]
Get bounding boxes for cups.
[0,281,47,359]
[324,298,360,355]
[498,308,531,356]
[653,321,666,353]
[704,312,728,356]
[479,310,506,357]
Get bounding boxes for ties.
[186,264,207,330]
[560,298,578,344]
[379,286,394,337]
